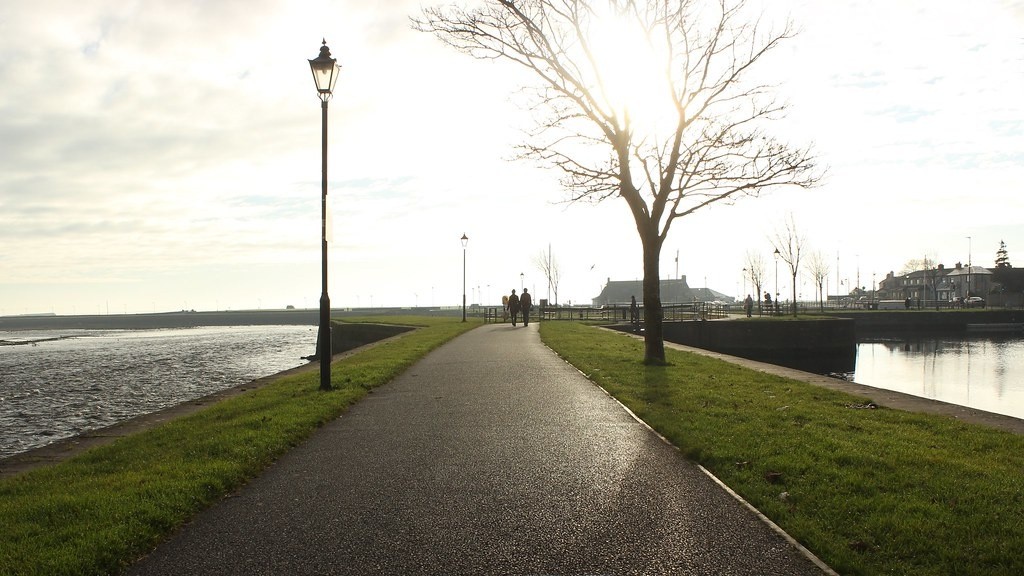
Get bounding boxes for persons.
[630,295,639,323]
[905,296,970,311]
[579,306,584,318]
[518,288,531,326]
[744,294,753,318]
[507,289,519,327]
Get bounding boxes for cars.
[949,297,963,306]
[963,297,985,308]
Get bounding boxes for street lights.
[307,38,342,394]
[742,268,747,300]
[461,233,468,322]
[520,273,525,290]
[773,248,780,317]
[965,236,971,300]
